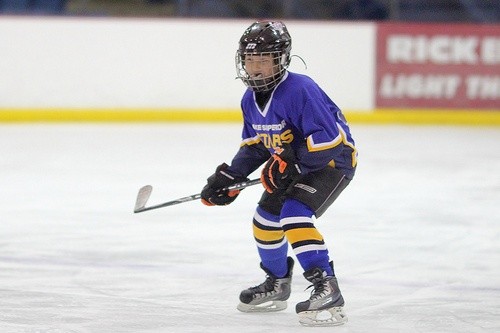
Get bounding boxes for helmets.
[235,20,291,92]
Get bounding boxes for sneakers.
[236,256,295,313]
[296,260,349,328]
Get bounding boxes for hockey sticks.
[134,164,288,214]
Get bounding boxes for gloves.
[200,163,250,205]
[260,143,302,198]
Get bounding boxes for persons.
[200,19,358,325]
